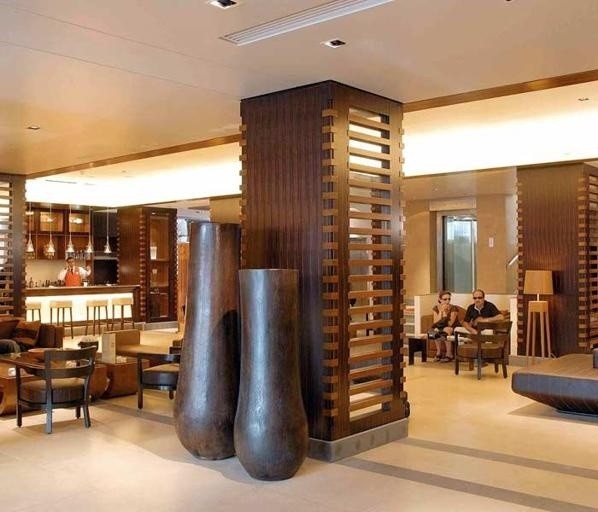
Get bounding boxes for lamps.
[523,269,556,367]
[23,202,112,254]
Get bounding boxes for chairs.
[0,341,98,434]
[455,322,512,379]
[102,327,182,410]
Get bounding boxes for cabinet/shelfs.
[115,207,176,322]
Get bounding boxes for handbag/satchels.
[427,331,448,340]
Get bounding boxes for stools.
[408,334,426,363]
[85,298,136,336]
[26,300,74,339]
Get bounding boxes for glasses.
[473,297,483,299]
[443,298,451,301]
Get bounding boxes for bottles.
[28,277,34,288]
[68,249,84,259]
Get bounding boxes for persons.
[428,289,506,365]
[56,256,94,287]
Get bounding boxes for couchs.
[0,315,65,354]
[421,314,510,359]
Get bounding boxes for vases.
[174,221,305,483]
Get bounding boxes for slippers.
[434,355,454,363]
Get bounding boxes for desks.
[0,342,149,416]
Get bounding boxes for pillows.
[1,314,41,348]
